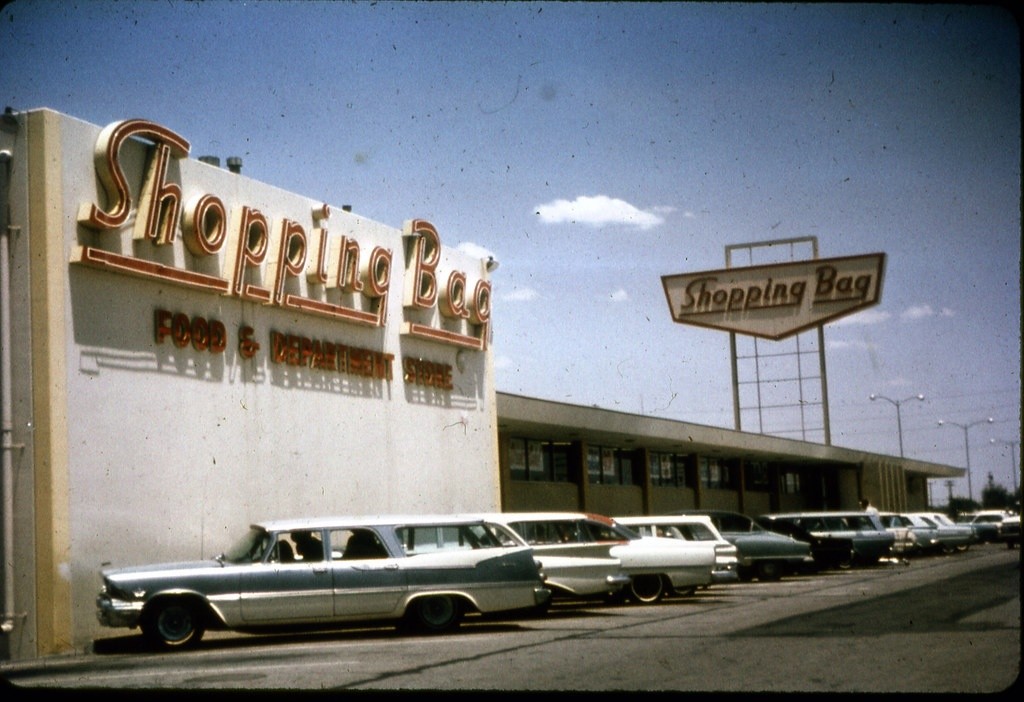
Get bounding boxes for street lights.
[990,438,1019,492]
[870,392,924,457]
[938,417,995,500]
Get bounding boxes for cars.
[610,513,740,597]
[94,513,555,653]
[396,511,718,607]
[676,509,1023,582]
